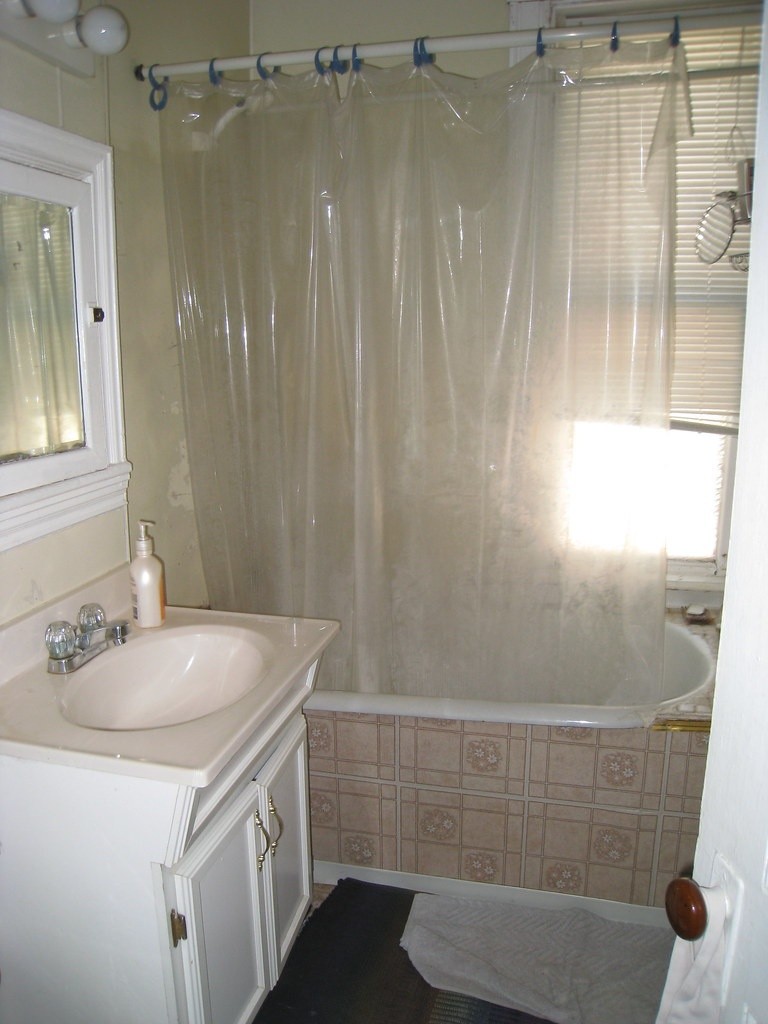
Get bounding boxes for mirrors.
[0,191,86,465]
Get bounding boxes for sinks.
[60,624,273,732]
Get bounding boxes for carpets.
[252,877,677,1024]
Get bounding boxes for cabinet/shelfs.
[0,713,313,1024]
[0,108,132,552]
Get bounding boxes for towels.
[399,890,680,1024]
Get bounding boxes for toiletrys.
[130,519,168,627]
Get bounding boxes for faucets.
[45,602,131,674]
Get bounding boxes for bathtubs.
[304,608,722,931]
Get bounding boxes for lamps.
[0,0,128,79]
